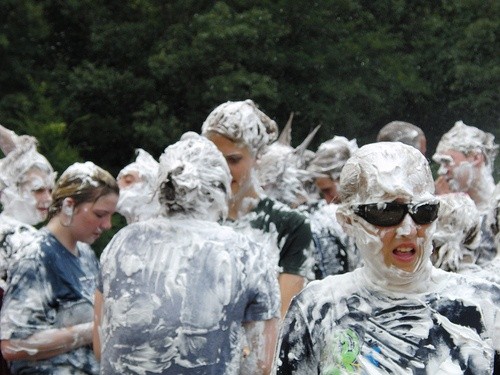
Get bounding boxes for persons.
[255,112,500,286]
[271,142,500,375]
[115,148,161,225]
[93,131,282,375]
[201,99,315,320]
[0,161,120,375]
[0,124,55,303]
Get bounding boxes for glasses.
[349,198,439,228]
